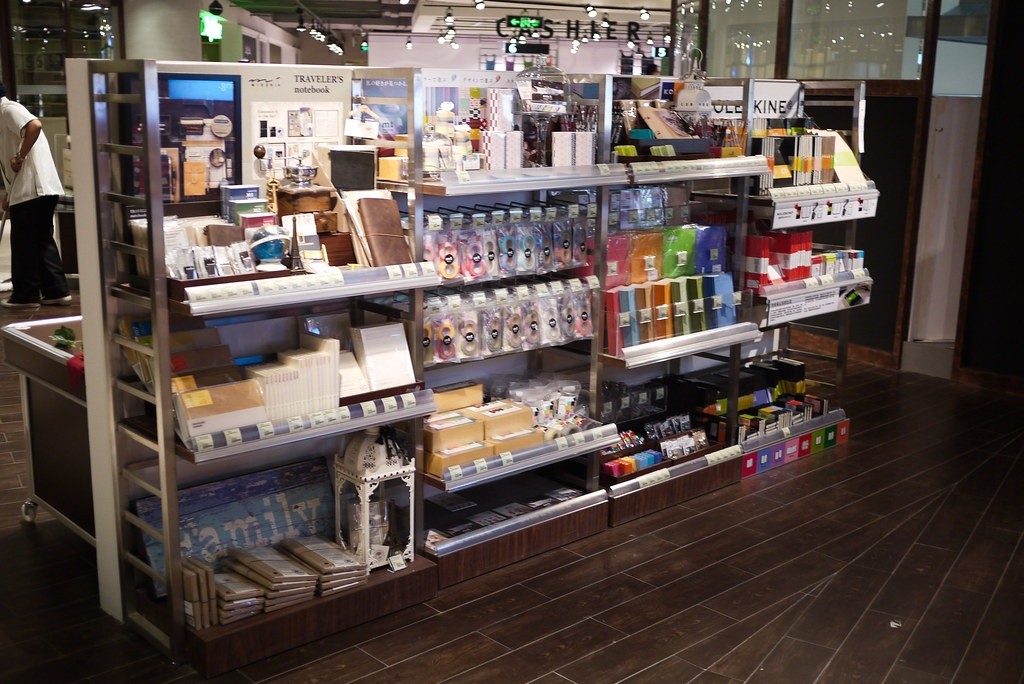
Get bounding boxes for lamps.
[361,40,368,47]
[640,7,647,13]
[646,37,654,44]
[593,31,601,38]
[437,27,456,45]
[663,34,671,41]
[308,18,344,56]
[510,29,538,43]
[473,0,485,10]
[444,13,454,23]
[601,18,609,27]
[640,11,650,20]
[587,9,597,18]
[406,41,413,48]
[627,39,636,48]
[585,4,593,12]
[295,9,307,32]
[581,35,588,42]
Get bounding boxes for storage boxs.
[473,403,533,440]
[428,441,495,479]
[423,417,485,452]
[459,396,512,417]
[431,380,483,413]
[486,428,544,457]
[229,199,268,225]
[220,185,260,219]
[423,408,468,429]
[239,212,275,242]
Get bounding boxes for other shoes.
[39,293,72,306]
[0,297,41,307]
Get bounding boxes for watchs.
[16,151,27,162]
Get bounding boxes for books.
[117,150,415,630]
[604,99,867,445]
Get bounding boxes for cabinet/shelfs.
[65,57,880,680]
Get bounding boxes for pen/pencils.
[689,114,751,156]
[562,100,624,148]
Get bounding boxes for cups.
[483,132,507,170]
[489,89,513,133]
[552,132,575,166]
[575,132,596,166]
[506,131,523,168]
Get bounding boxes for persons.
[0,81,72,308]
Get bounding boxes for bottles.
[422,123,440,171]
